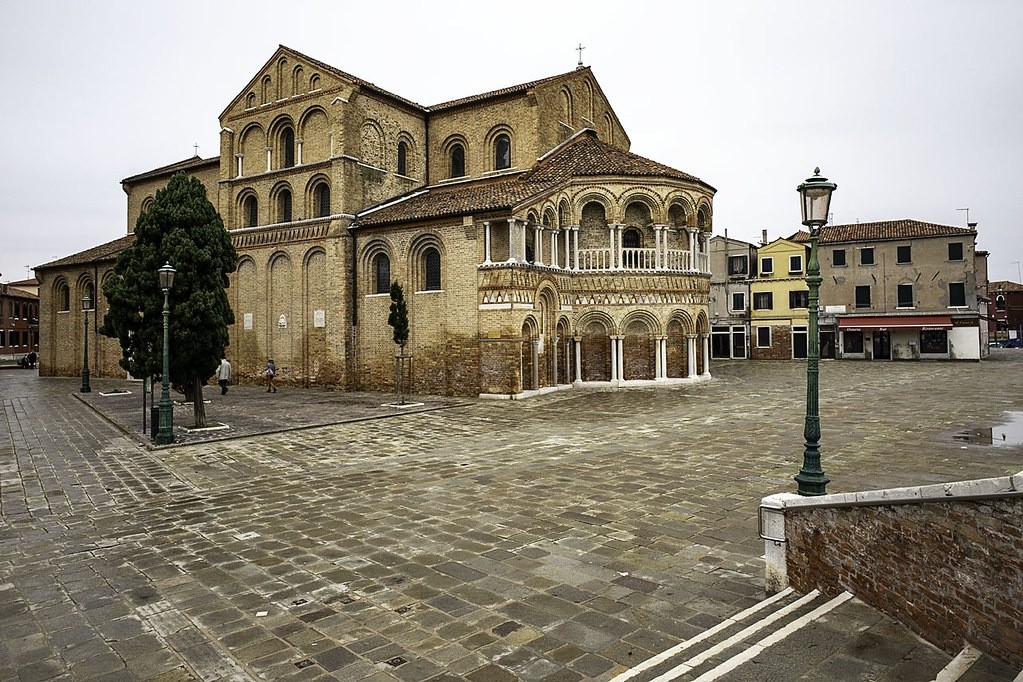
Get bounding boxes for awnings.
[838,315,954,332]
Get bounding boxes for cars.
[990,339,1023,350]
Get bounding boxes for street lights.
[155,261,177,445]
[794,165,838,496]
[1010,261,1022,283]
[956,208,969,225]
[81,294,92,393]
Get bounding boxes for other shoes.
[222,388,228,395]
[273,388,277,393]
[267,390,271,392]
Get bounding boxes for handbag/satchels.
[266,368,273,377]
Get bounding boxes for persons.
[22,350,37,370]
[216,357,231,395]
[264,359,277,392]
